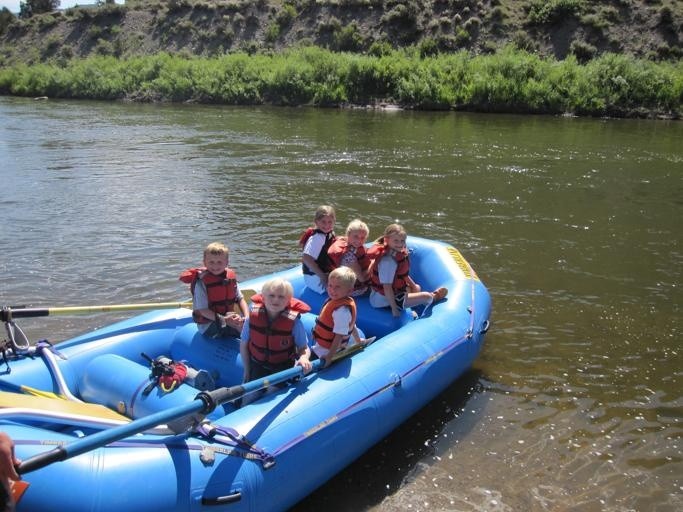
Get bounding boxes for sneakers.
[429,288,448,301]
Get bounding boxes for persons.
[191,240,250,340]
[237,275,313,408]
[308,265,368,370]
[0,432,21,511]
[300,205,336,295]
[368,223,448,319]
[325,217,375,298]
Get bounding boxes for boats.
[0,234,490,511]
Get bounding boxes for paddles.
[0,289,257,322]
[16,336,377,473]
